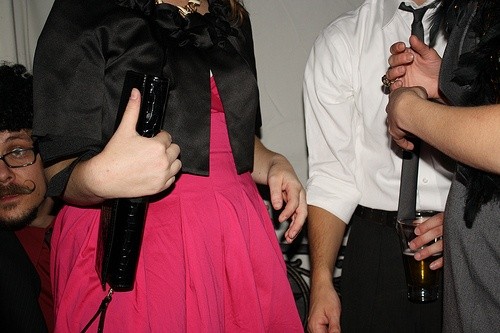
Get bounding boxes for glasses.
[1,144,38,167]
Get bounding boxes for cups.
[397,209,444,302]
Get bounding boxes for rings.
[382,75,398,87]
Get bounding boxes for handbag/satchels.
[96,70,167,292]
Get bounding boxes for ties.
[396,0,439,229]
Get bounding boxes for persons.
[0,64,61,333]
[382,0,500,333]
[33,0,308,333]
[303,0,457,333]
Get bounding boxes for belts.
[357,205,398,227]
